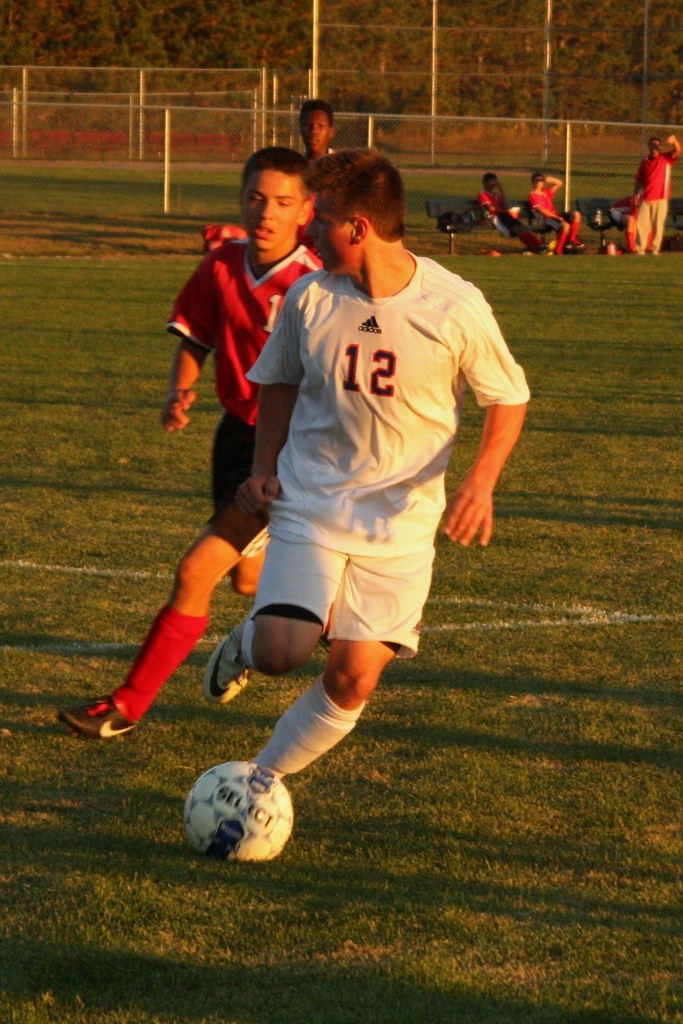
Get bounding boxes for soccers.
[181,759,296,862]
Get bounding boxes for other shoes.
[567,239,585,250]
[637,247,660,256]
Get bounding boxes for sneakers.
[201,609,253,708]
[53,695,138,741]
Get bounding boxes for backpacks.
[438,212,473,233]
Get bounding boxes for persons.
[60,100,530,778]
[477,173,585,255]
[609,135,681,254]
